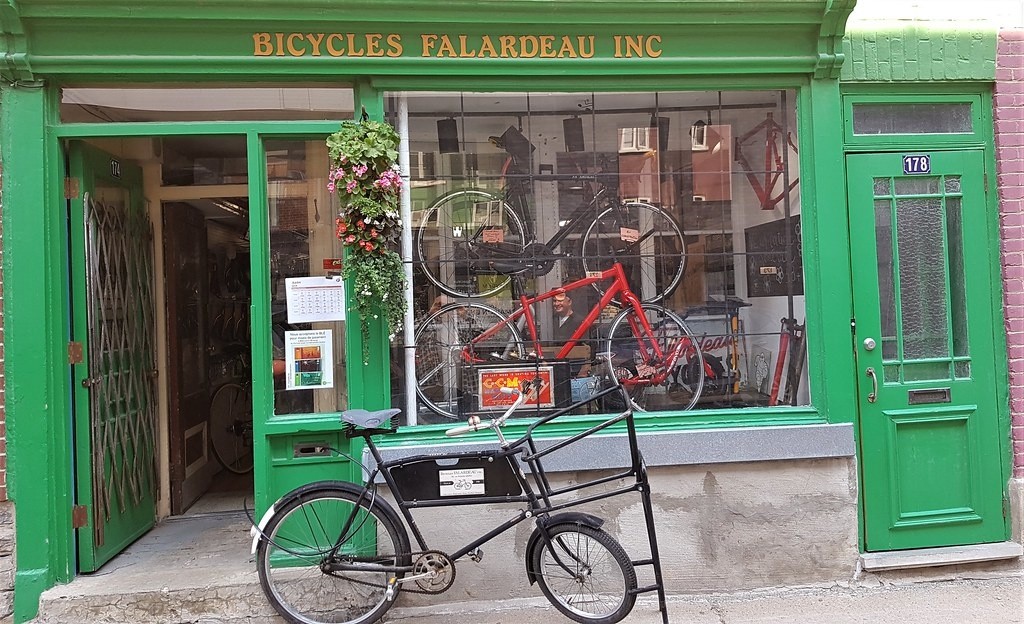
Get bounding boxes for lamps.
[498,117,536,161]
[688,107,725,154]
[437,117,460,155]
[649,112,670,153]
[563,115,584,155]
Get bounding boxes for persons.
[271,317,312,415]
[415,295,485,398]
[551,288,595,380]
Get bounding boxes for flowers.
[327,124,407,367]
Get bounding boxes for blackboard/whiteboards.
[744,214,804,297]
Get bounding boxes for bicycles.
[404,228,705,422]
[208,348,256,475]
[415,136,688,308]
[250,381,638,624]
